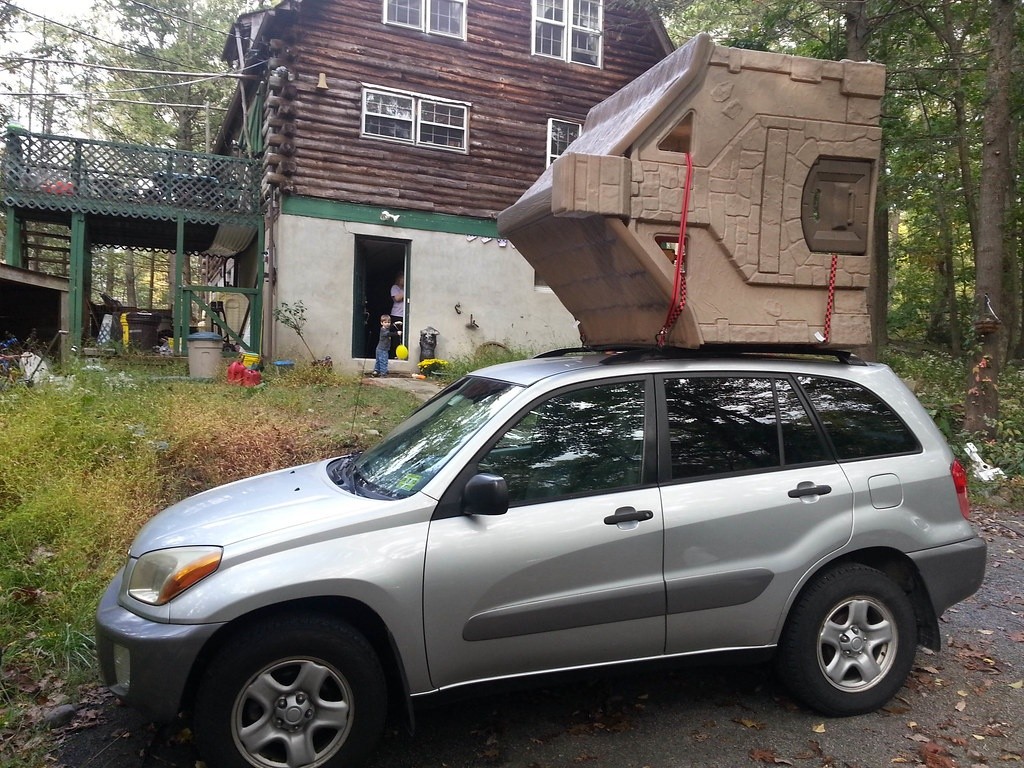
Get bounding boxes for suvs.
[94,336,990,768]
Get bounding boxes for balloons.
[396,344,408,360]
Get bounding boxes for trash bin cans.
[121,311,161,354]
[186,332,223,378]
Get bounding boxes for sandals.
[380,372,387,378]
[371,371,379,377]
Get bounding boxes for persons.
[372,315,402,378]
[390,271,404,359]
